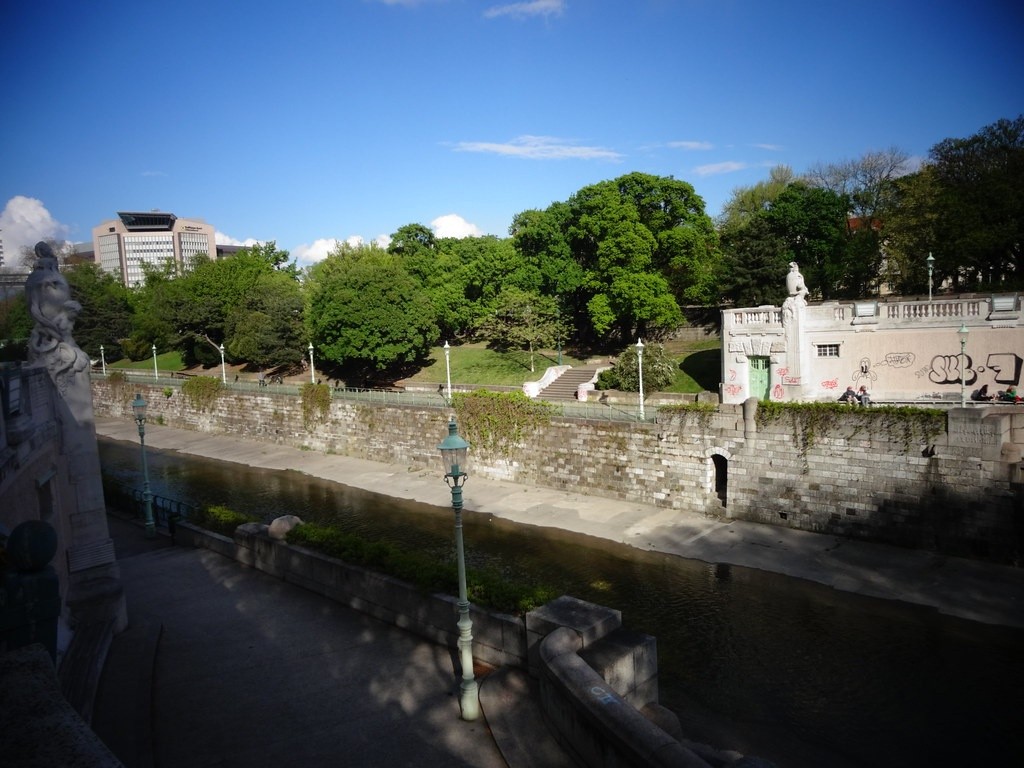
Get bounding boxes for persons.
[839,386,858,407]
[45,300,87,384]
[858,385,869,408]
[258,371,265,386]
[1001,385,1021,401]
[975,385,993,401]
[880,297,887,302]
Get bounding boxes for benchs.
[838,401,1023,406]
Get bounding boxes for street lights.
[444,340,452,399]
[152,345,159,381]
[957,323,969,407]
[554,296,562,365]
[635,338,646,420]
[927,252,936,302]
[438,423,484,718]
[100,346,106,376]
[131,393,154,529]
[524,305,534,372]
[219,344,226,386]
[307,342,315,384]
